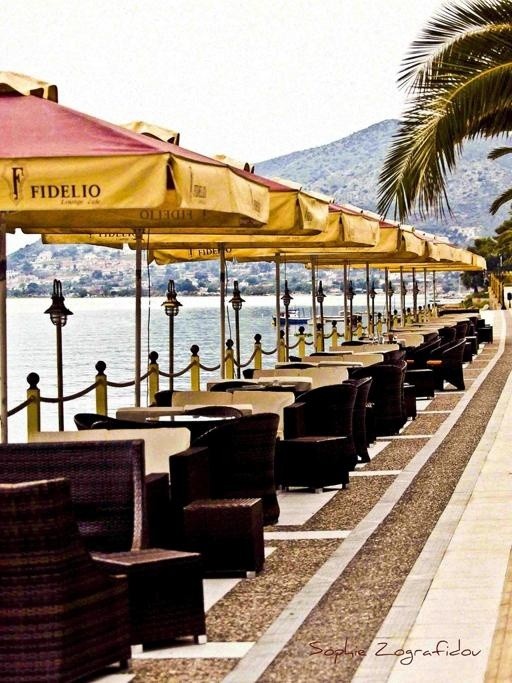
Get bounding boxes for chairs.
[0,312,492,683]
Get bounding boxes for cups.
[368,332,394,345]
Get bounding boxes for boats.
[272,309,345,325]
